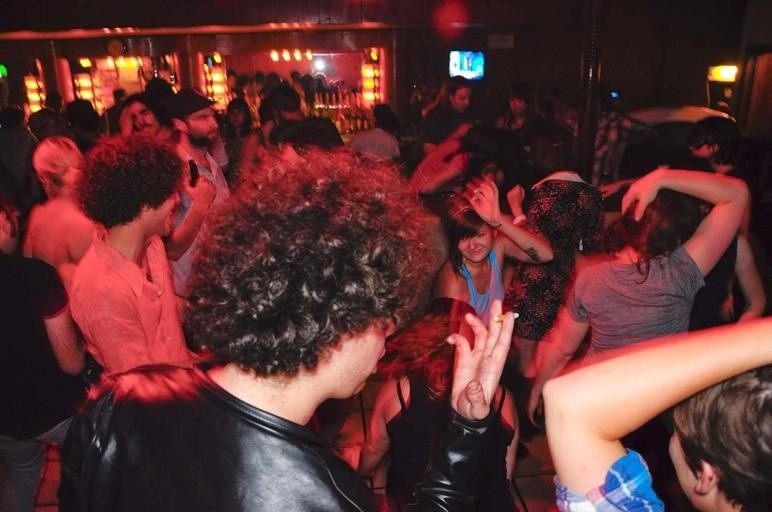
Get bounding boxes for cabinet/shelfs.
[309,86,370,137]
[170,73,178,95]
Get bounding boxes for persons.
[59,156,514,512]
[0,70,349,512]
[543,318,772,511]
[327,78,772,478]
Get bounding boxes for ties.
[448,49,486,80]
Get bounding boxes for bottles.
[165,87,212,116]
